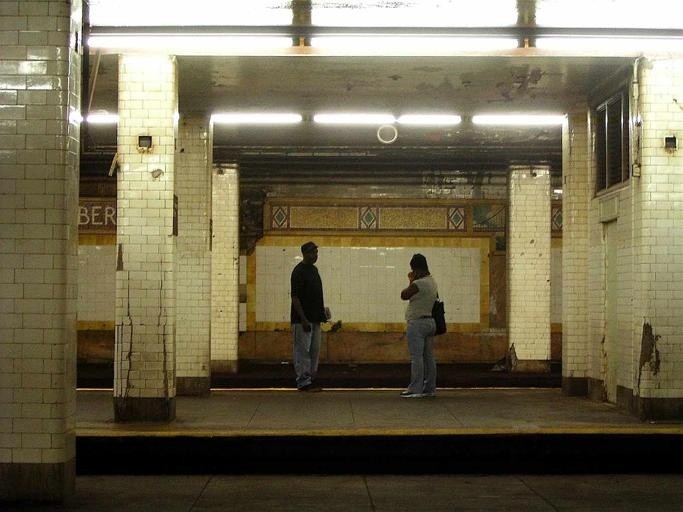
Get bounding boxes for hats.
[301,242,318,254]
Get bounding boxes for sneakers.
[423,390,437,398]
[298,385,322,393]
[399,390,424,398]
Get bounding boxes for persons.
[400,254,439,398]
[289,241,327,392]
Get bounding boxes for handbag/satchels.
[431,301,446,336]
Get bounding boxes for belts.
[419,315,430,320]
